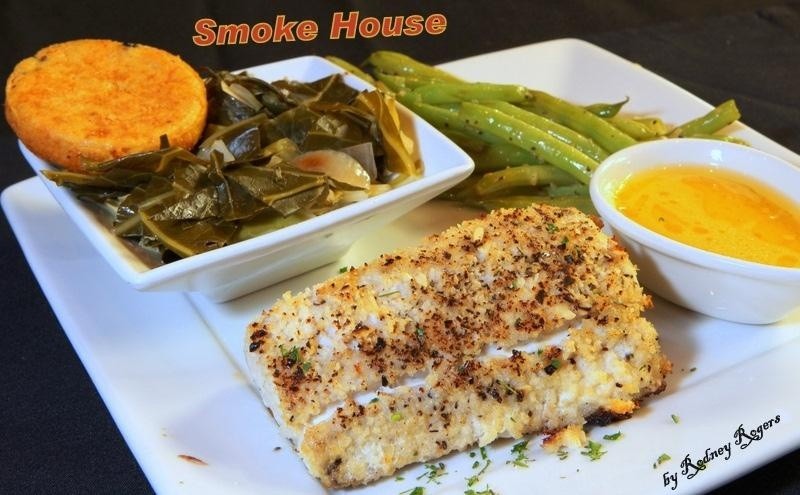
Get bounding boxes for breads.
[245,202,674,490]
[5,37,208,173]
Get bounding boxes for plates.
[0,35,799,494]
[18,53,475,306]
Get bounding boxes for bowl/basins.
[585,133,798,329]
